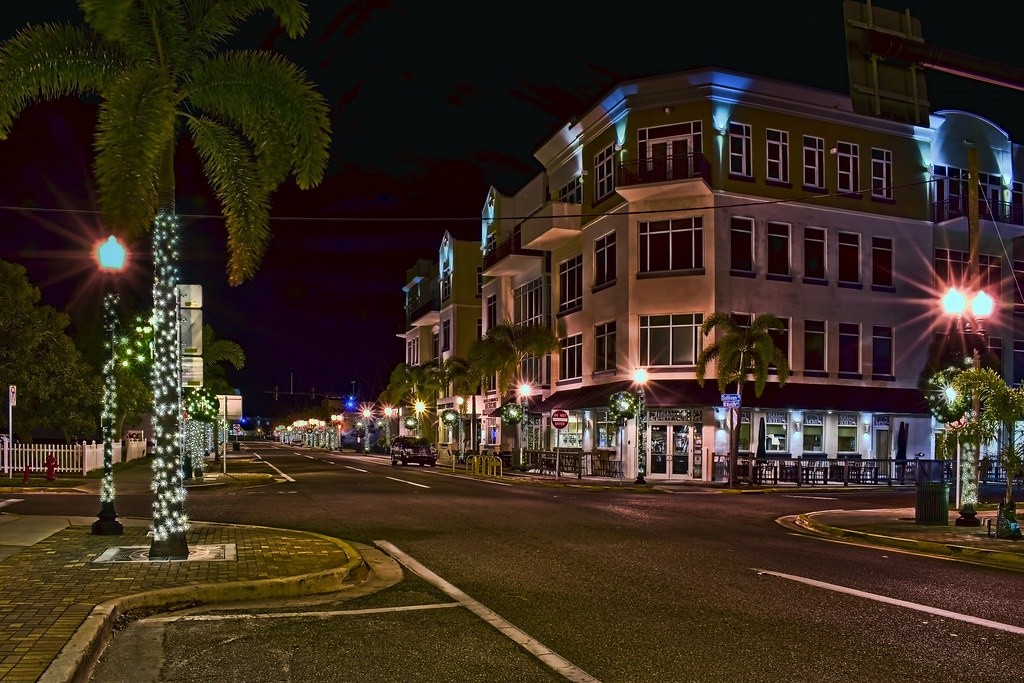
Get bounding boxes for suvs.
[391,435,439,473]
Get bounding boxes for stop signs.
[551,409,569,430]
[233,424,240,433]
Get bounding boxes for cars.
[290,439,303,447]
[341,425,379,452]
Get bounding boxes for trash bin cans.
[233,442,240,451]
[913,481,953,525]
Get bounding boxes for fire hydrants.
[43,455,57,480]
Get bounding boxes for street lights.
[635,369,646,484]
[937,282,997,500]
[92,234,124,539]
[521,385,528,470]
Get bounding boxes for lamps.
[582,170,588,175]
[831,148,837,153]
[615,144,621,150]
[719,130,725,135]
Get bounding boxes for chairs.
[448,449,511,467]
